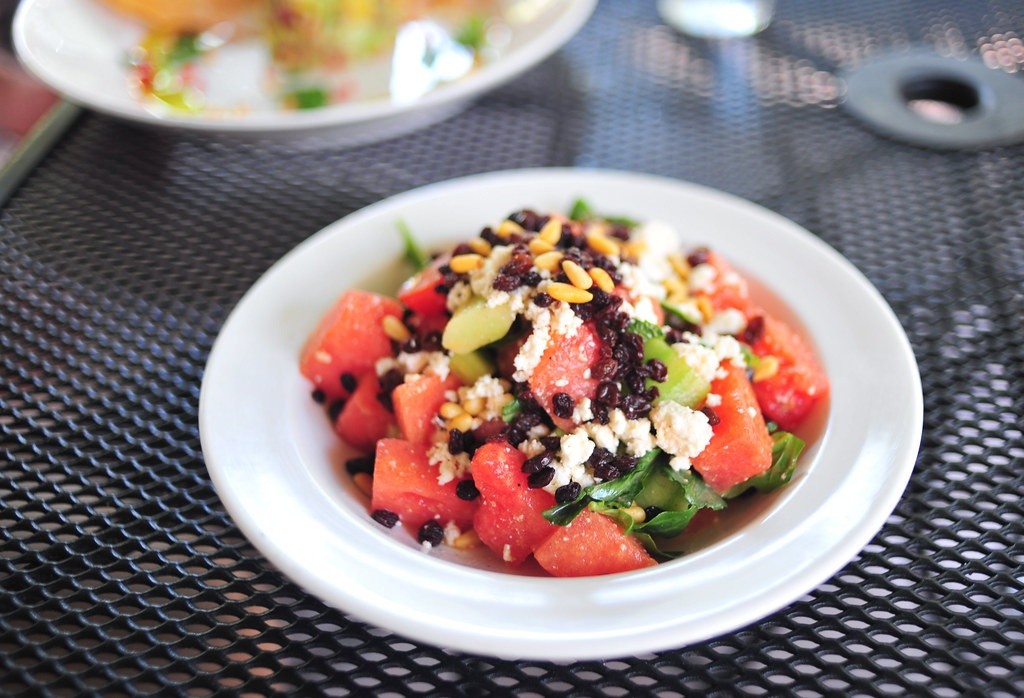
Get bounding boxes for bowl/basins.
[10,1,597,151]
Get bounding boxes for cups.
[658,2,775,38]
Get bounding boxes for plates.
[198,168,924,663]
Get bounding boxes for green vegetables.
[156,23,488,109]
[395,200,809,560]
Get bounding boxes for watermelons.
[303,256,824,579]
